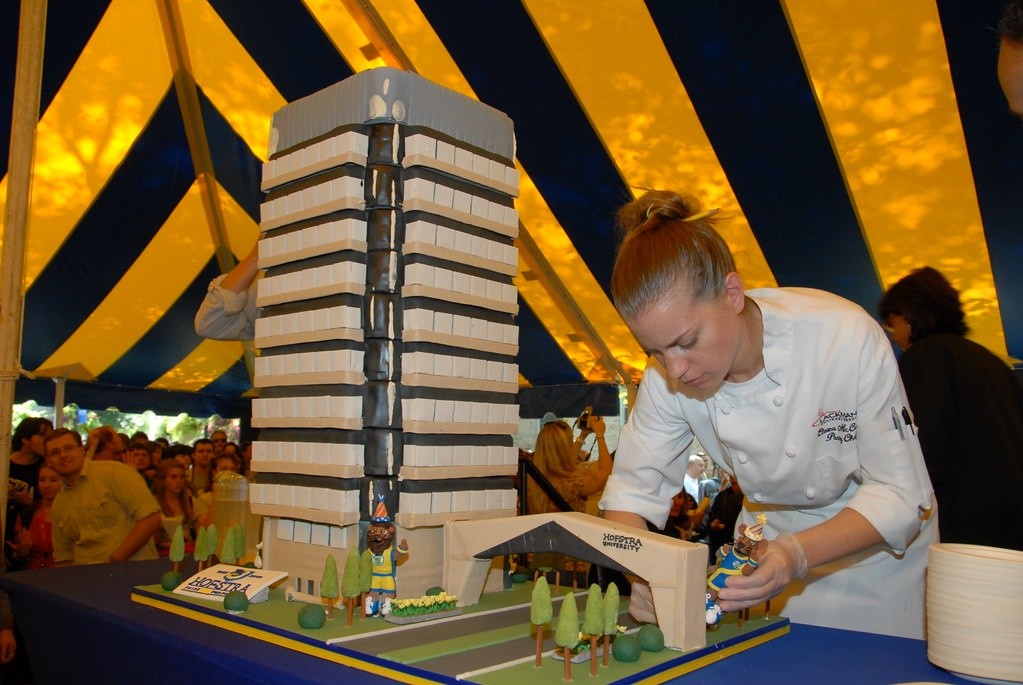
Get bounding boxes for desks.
[0,557,972,685]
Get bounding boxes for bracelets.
[108,553,116,563]
[597,437,604,440]
[576,437,585,444]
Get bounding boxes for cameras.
[577,406,600,431]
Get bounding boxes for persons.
[194,227,263,339]
[876,267,1022,552]
[7,418,252,565]
[598,187,939,641]
[0,573,17,664]
[514,406,745,561]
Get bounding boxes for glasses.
[36,431,47,439]
[882,314,896,333]
[213,438,227,443]
[45,443,82,458]
[544,421,566,431]
